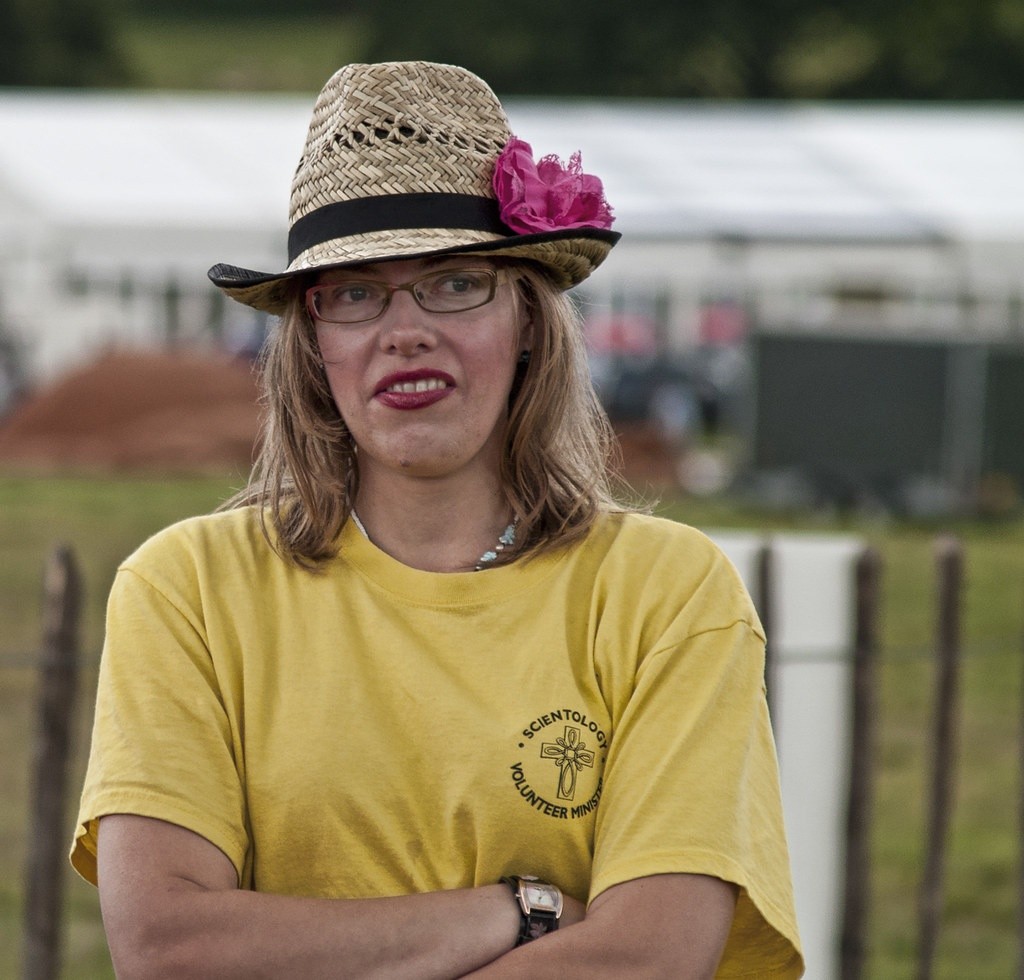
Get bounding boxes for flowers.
[489,133,619,234]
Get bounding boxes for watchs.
[498,874,564,947]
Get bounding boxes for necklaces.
[474,505,520,572]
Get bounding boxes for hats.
[205,62,620,318]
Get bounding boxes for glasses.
[295,261,526,329]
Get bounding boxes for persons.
[67,64,811,980]
